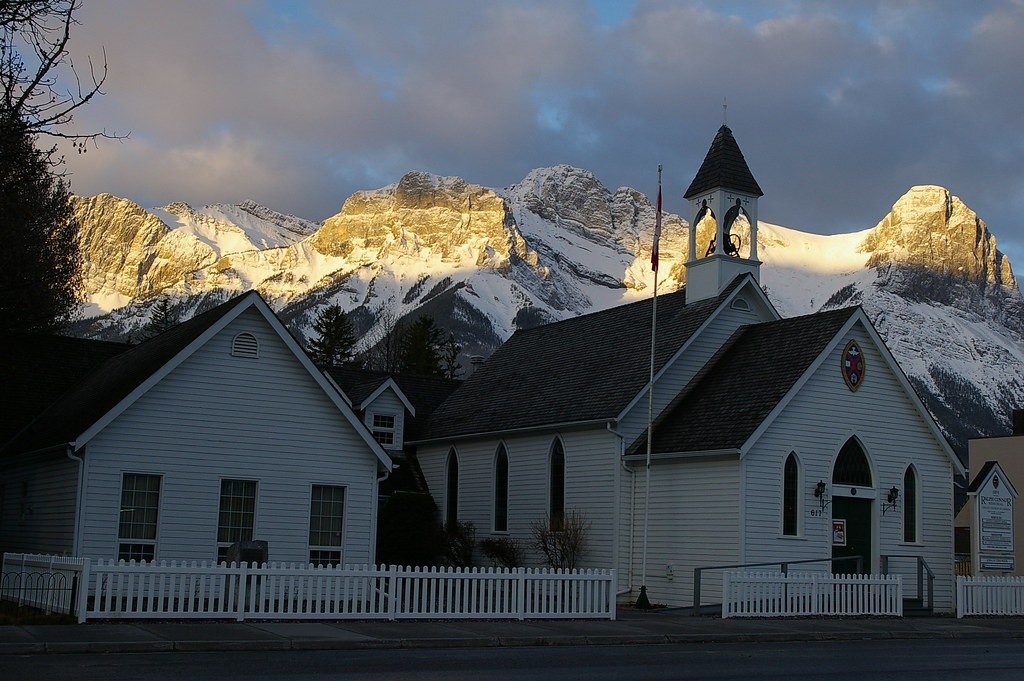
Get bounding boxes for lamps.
[883,486,899,516]
[814,480,826,507]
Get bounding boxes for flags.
[651,185,662,272]
